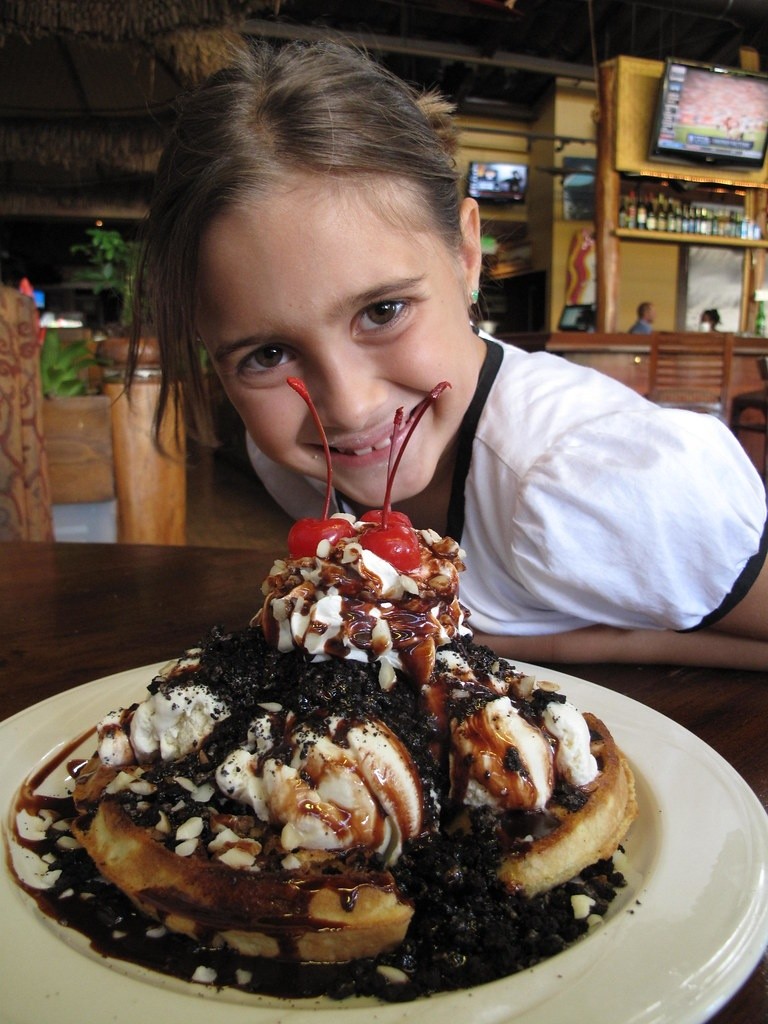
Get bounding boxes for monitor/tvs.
[647,56,768,172]
[466,161,529,205]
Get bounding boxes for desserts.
[72,379,636,961]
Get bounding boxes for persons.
[699,307,721,332]
[499,169,523,192]
[478,168,499,188]
[676,71,768,132]
[724,117,744,141]
[119,36,768,675]
[629,302,655,336]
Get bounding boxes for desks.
[0,541,768,814]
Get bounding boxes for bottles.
[618,180,761,239]
[755,301,765,337]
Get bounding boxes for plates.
[1,657,768,1024]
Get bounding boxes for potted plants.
[75,229,165,368]
[36,334,115,504]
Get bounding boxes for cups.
[699,321,711,333]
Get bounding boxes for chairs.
[646,334,732,428]
[0,285,54,543]
[736,356,768,440]
